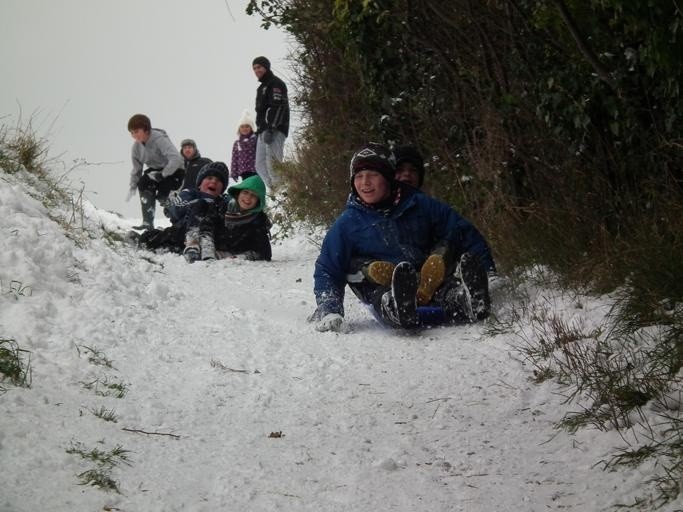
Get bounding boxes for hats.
[350,143,396,186]
[252,56,270,70]
[196,162,229,194]
[235,110,255,136]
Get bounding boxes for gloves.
[227,254,248,262]
[315,312,346,332]
[264,125,278,145]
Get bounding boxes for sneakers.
[183,231,216,260]
[362,247,492,330]
[132,225,154,231]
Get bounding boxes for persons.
[229,109,257,184]
[251,56,290,198]
[123,113,186,230]
[178,136,212,191]
[211,172,272,263]
[309,142,497,334]
[162,157,229,264]
[345,141,464,308]
